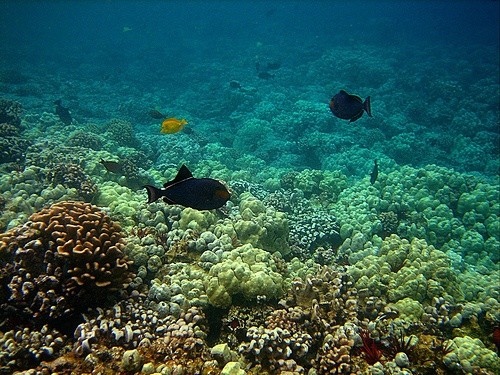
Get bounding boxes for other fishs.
[159,118,188,134]
[98,7,326,94]
[54,99,72,125]
[329,89,373,125]
[141,164,232,210]
[369,159,379,185]
[99,158,124,174]
